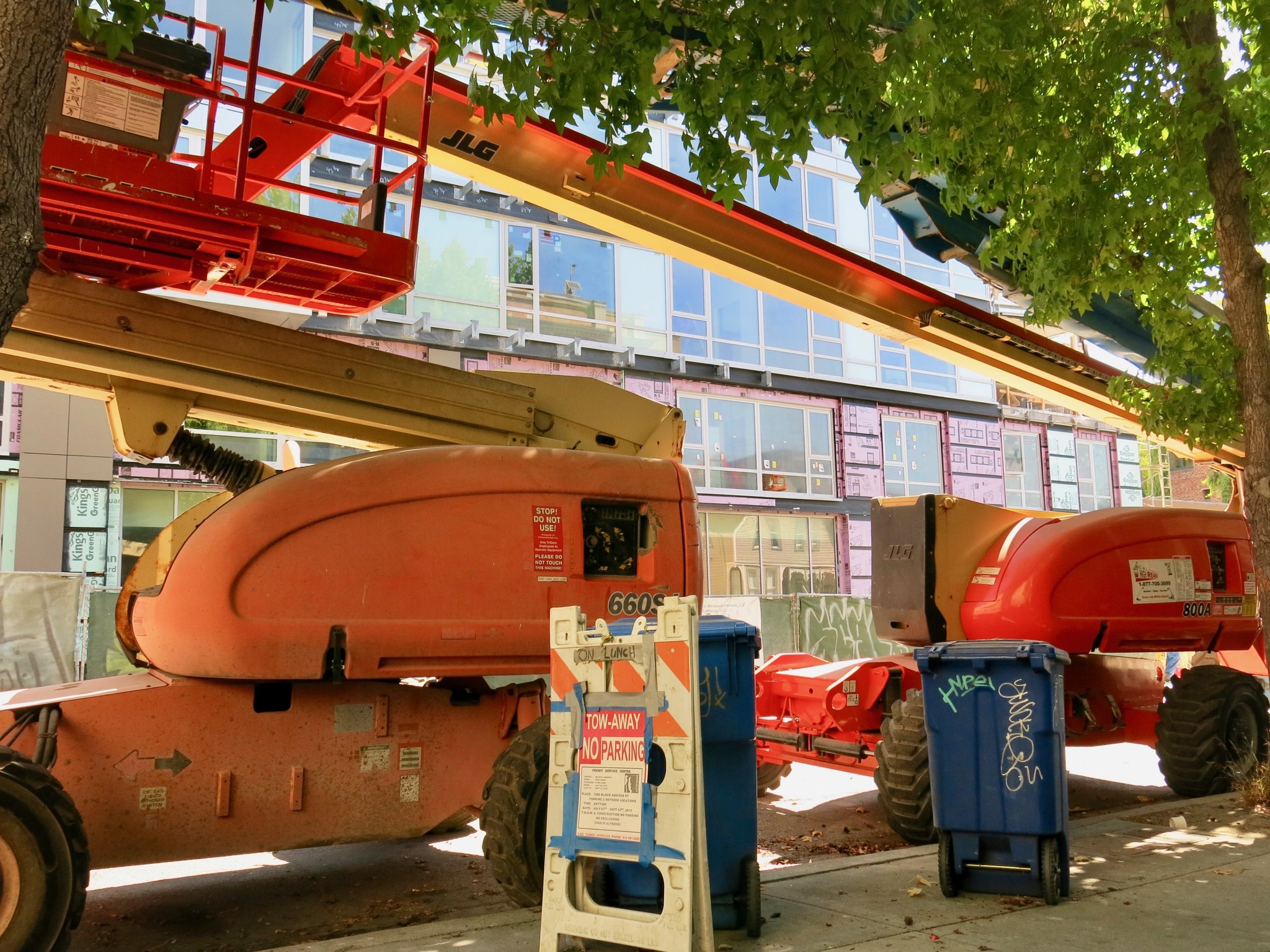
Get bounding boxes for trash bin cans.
[595,611,763,937]
[913,637,1074,904]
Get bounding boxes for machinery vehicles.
[0,263,705,952]
[0,0,1270,952]
